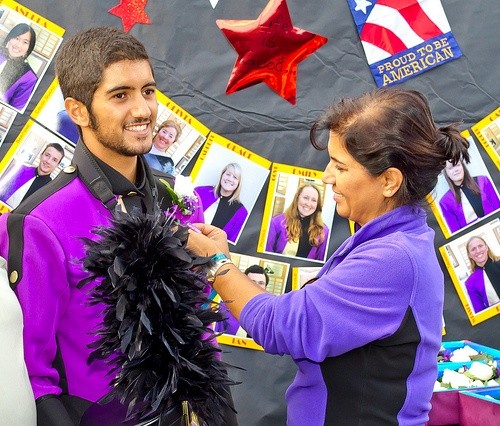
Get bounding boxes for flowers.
[160,174,200,215]
[431,342,500,391]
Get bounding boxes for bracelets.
[202,254,235,284]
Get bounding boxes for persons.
[193,163,248,243]
[265,183,330,261]
[214,264,270,339]
[0,143,64,209]
[437,158,500,233]
[464,235,500,313]
[144,120,181,177]
[172,88,470,426]
[0,27,236,426]
[0,23,37,111]
